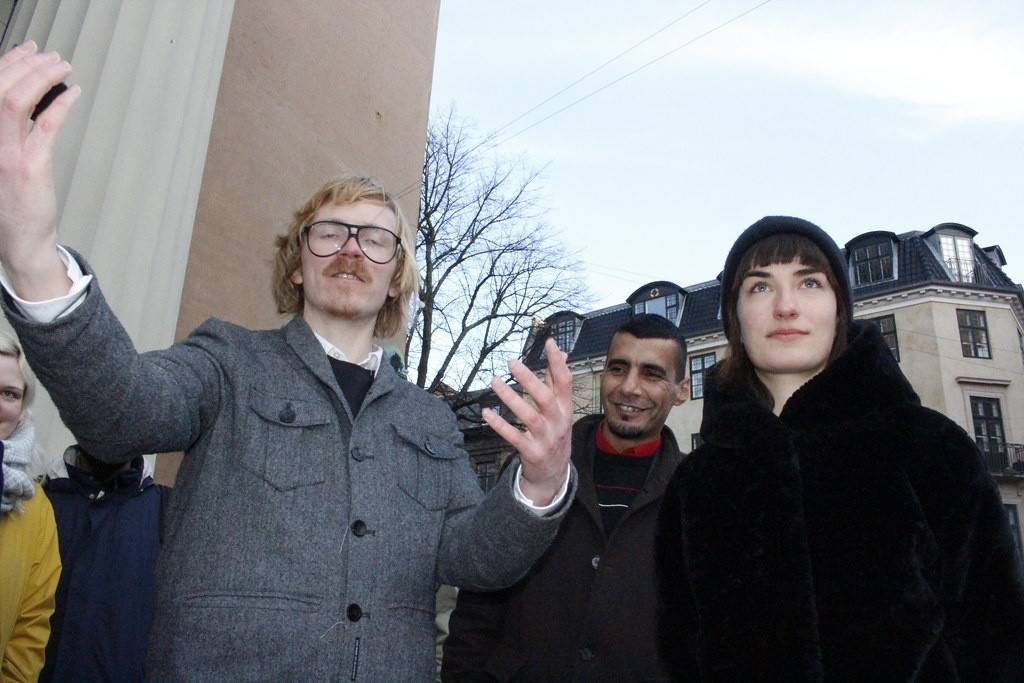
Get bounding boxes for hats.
[721,215,855,350]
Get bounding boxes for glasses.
[305,220,405,265]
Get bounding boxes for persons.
[1,38,574,682]
[441,312,692,683]
[0,315,62,683]
[42,441,173,683]
[655,215,1023,681]
